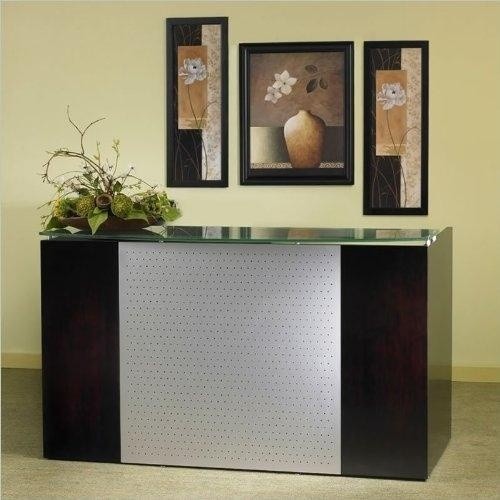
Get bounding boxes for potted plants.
[35,107,184,237]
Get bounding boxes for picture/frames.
[363,39,429,216]
[238,41,354,186]
[166,16,229,188]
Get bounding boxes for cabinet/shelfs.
[38,223,453,482]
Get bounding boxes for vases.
[58,217,130,234]
